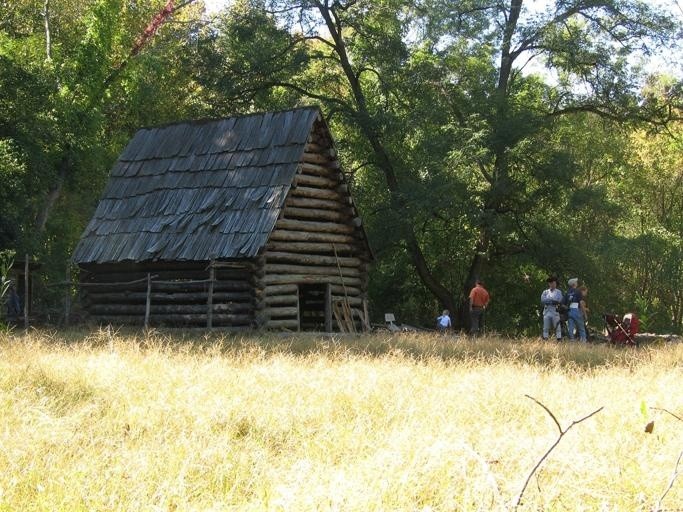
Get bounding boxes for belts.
[472,306,482,308]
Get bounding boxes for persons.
[436,309,452,332]
[541,277,589,342]
[468,279,490,329]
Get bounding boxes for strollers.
[600,311,641,348]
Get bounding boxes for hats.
[568,277,578,286]
[546,277,557,282]
